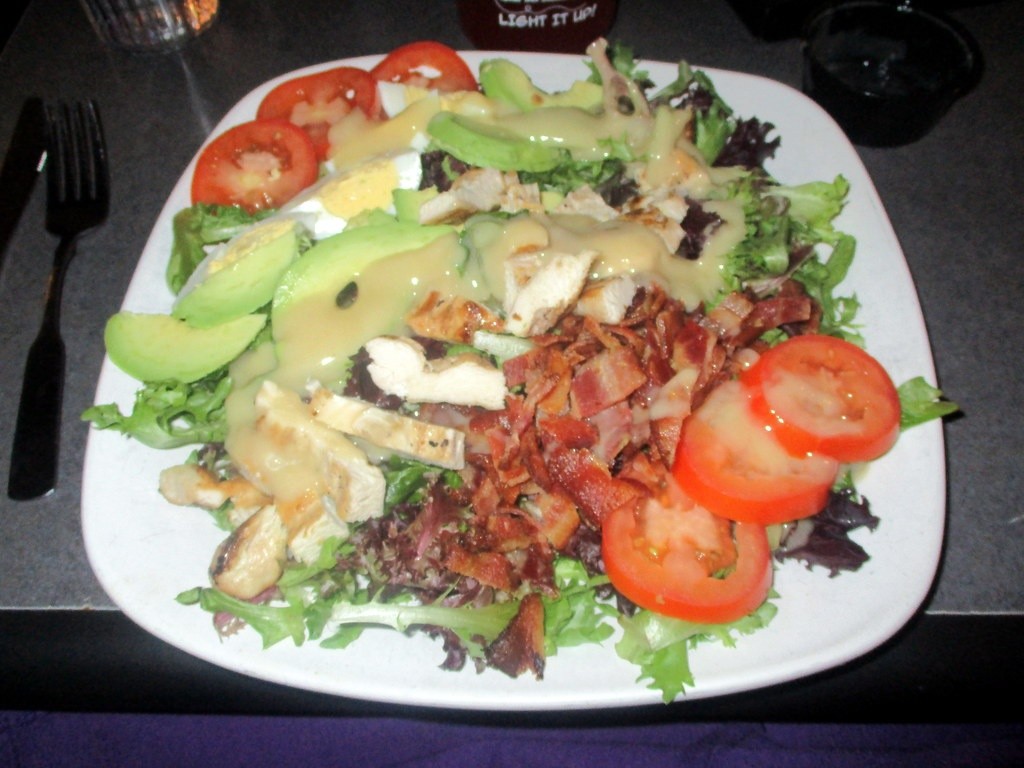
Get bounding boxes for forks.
[6,94,112,500]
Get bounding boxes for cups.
[457,1,616,57]
[79,0,218,54]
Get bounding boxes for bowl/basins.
[800,3,989,153]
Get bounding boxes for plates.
[78,51,948,713]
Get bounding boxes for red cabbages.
[78,32,962,703]
